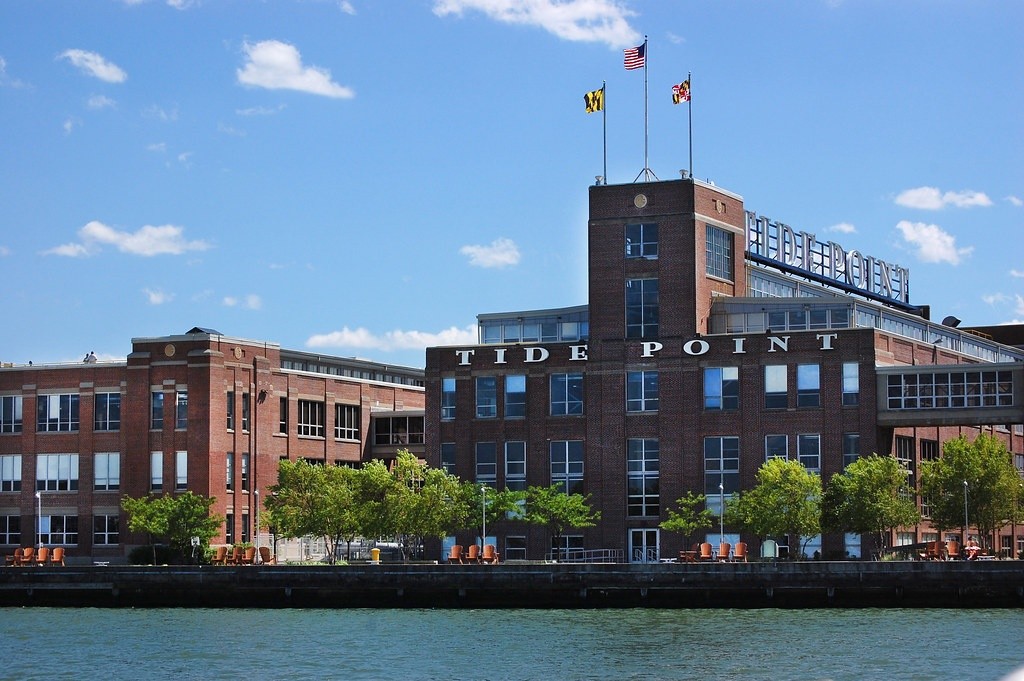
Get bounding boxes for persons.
[83,351,97,364]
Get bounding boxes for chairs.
[51,547,66,566]
[481,545,499,565]
[447,545,465,564]
[34,547,50,566]
[679,542,699,562]
[465,545,481,564]
[733,542,748,562]
[259,547,277,564]
[699,543,715,562]
[211,547,256,566]
[716,543,732,562]
[5,547,35,566]
[914,541,982,561]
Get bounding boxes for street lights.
[718,481,725,544]
[253,490,260,564]
[480,487,487,546]
[963,478,968,541]
[34,492,42,549]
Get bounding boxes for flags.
[583,89,604,113]
[671,79,691,105]
[623,43,645,72]
